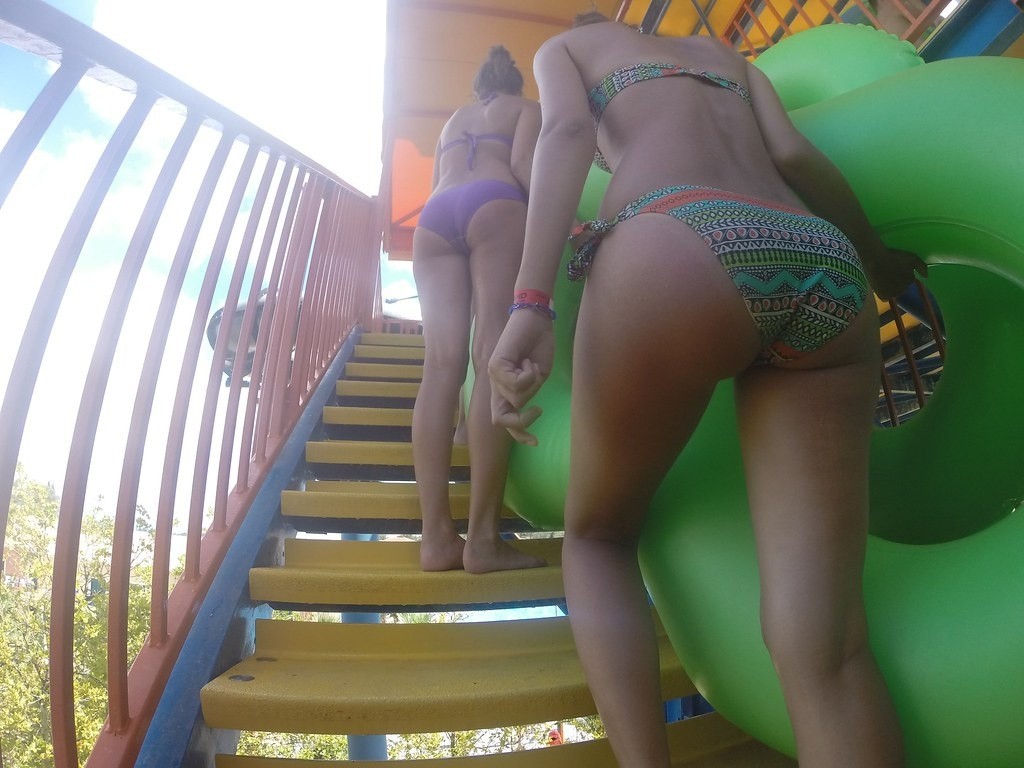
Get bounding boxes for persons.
[487,11,931,767]
[410,45,595,573]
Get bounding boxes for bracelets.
[568,221,589,243]
[509,287,557,322]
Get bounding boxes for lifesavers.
[460,25,1024,768]
